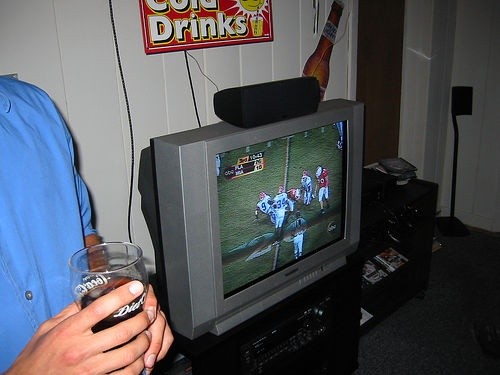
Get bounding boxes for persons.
[0,77,173,375]
[256,166,330,261]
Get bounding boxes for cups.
[68,241,148,375]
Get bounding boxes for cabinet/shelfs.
[153,168,439,375]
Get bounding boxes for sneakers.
[320,204,330,215]
[293,251,305,260]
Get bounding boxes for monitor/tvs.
[150,98,364,341]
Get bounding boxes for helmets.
[277,185,286,195]
[295,211,303,219]
[314,165,323,179]
[258,191,267,200]
[300,169,309,176]
